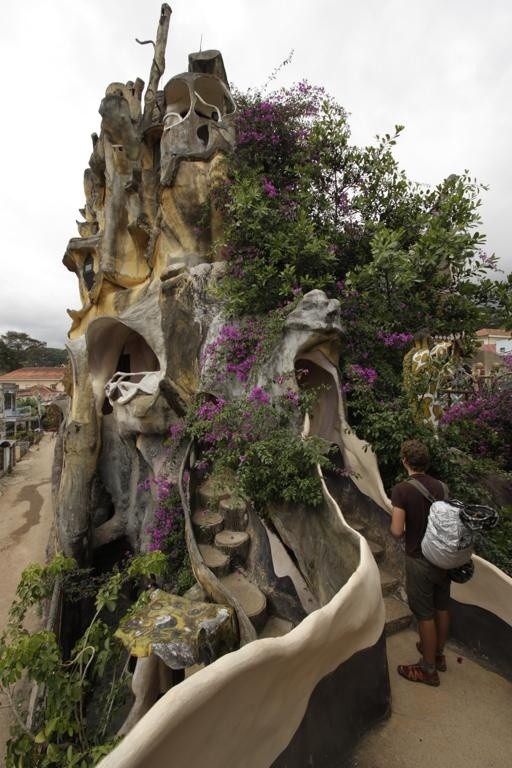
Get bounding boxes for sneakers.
[416,641,446,672]
[398,663,440,686]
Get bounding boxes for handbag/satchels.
[449,559,474,583]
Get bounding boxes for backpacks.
[404,477,474,569]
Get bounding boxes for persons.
[388,438,451,688]
